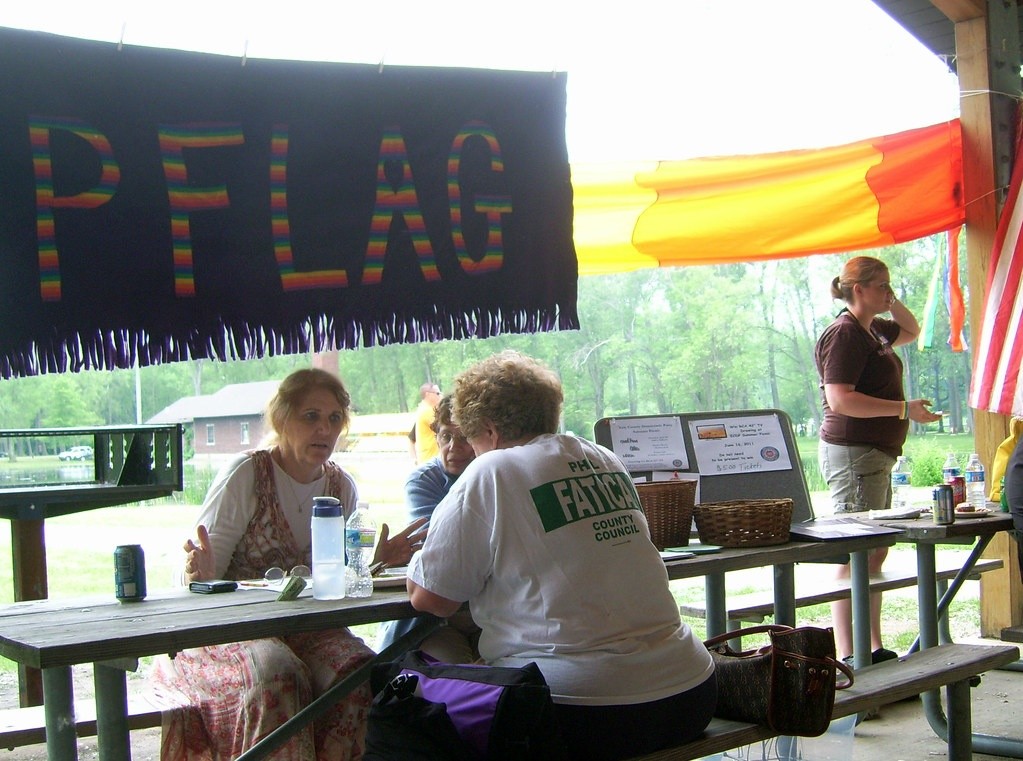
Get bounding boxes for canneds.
[931,484,955,524]
[999,476,1009,512]
[114,544,147,602]
[948,475,966,507]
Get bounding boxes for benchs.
[0,689,163,751]
[681,557,1004,652]
[639,642,1020,761]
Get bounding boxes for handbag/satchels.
[700,623,856,738]
[358,674,480,760]
[367,650,567,761]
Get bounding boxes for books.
[789,517,907,542]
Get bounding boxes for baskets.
[693,497,795,549]
[636,481,698,551]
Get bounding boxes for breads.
[955,502,975,512]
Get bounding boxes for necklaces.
[282,461,322,513]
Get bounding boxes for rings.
[411,546,413,552]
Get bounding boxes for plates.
[371,572,407,587]
[954,507,992,517]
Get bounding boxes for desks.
[0,503,1015,761]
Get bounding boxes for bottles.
[942,452,961,486]
[344,500,374,598]
[311,496,346,600]
[965,454,986,509]
[890,456,912,509]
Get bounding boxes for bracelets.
[890,296,896,307]
[900,401,908,420]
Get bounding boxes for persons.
[142,367,428,761]
[815,256,942,668]
[406,350,717,761]
[372,396,482,665]
[408,383,441,463]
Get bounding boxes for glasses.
[435,432,470,446]
[426,390,443,396]
[263,565,312,586]
